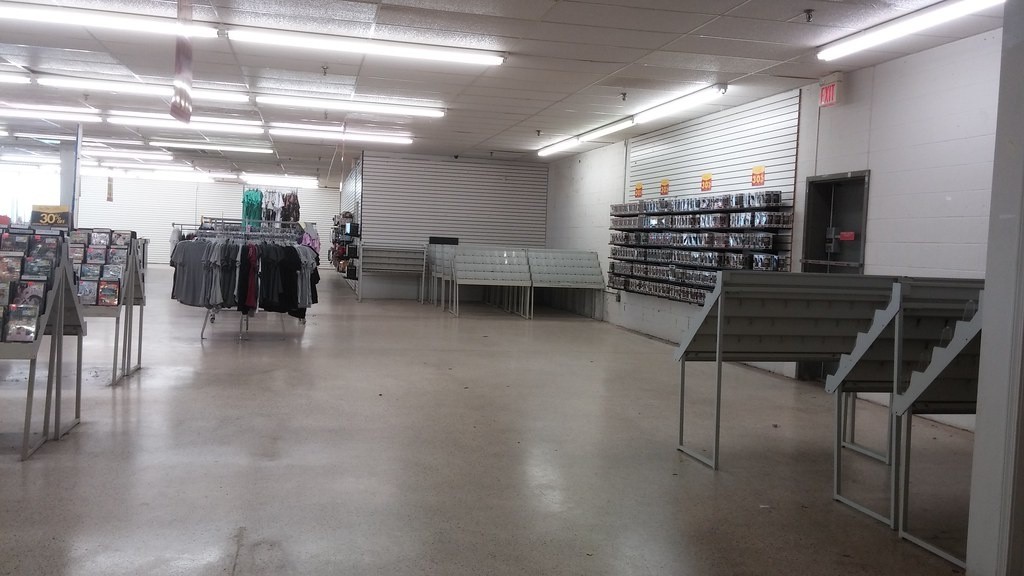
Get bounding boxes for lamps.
[0,0,1005,189]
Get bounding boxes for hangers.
[183,219,298,249]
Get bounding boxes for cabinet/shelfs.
[606,206,794,306]
